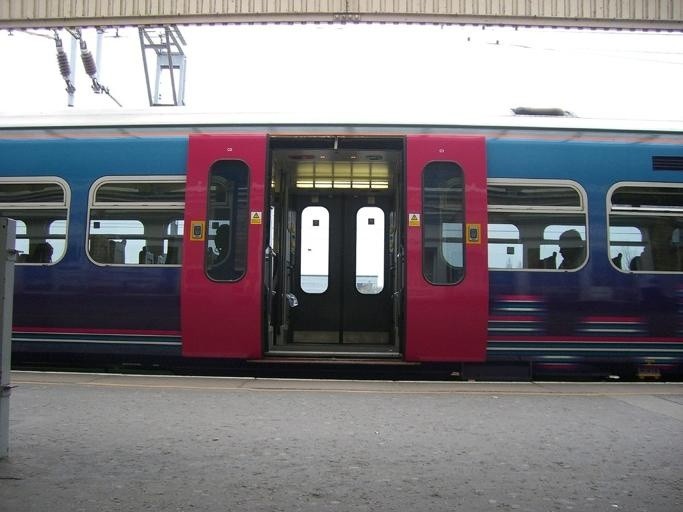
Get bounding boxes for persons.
[559,229,585,270]
[215,224,230,263]
[28,242,53,263]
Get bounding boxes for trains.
[0,127,681,381]
